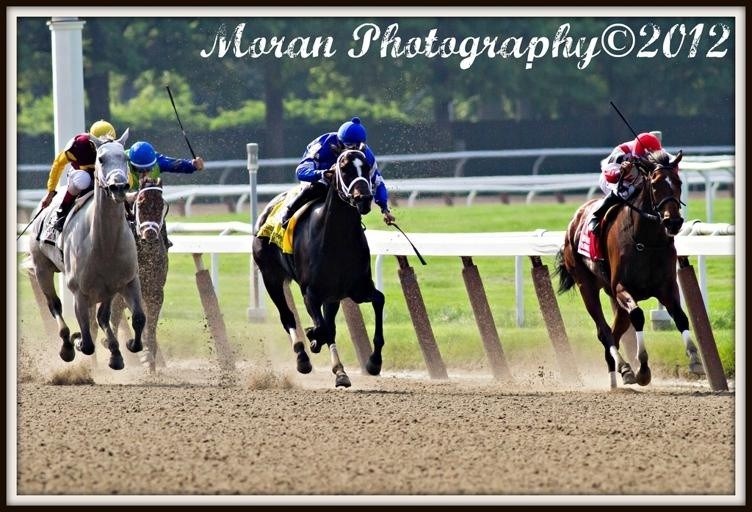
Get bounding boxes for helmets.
[336,117,367,149]
[90,119,116,140]
[632,133,661,158]
[129,141,157,169]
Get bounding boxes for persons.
[281,117,396,228]
[587,132,678,233]
[125,142,204,201]
[41,120,115,231]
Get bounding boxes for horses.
[88,171,168,376]
[28,128,146,370]
[554,149,706,390]
[252,142,385,387]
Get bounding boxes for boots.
[52,192,75,231]
[279,186,312,227]
[586,190,617,232]
